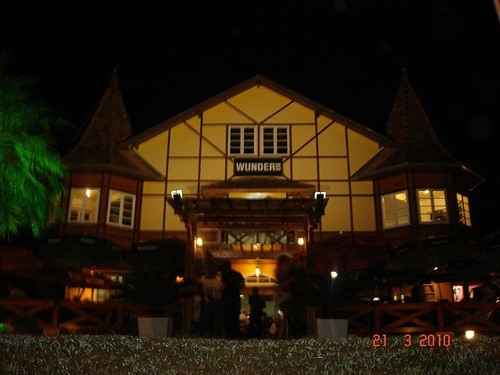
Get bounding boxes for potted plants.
[305,272,379,338]
[128,265,205,340]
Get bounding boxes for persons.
[197,262,225,338]
[222,261,245,339]
[278,255,307,339]
[249,288,266,339]
[364,281,500,337]
